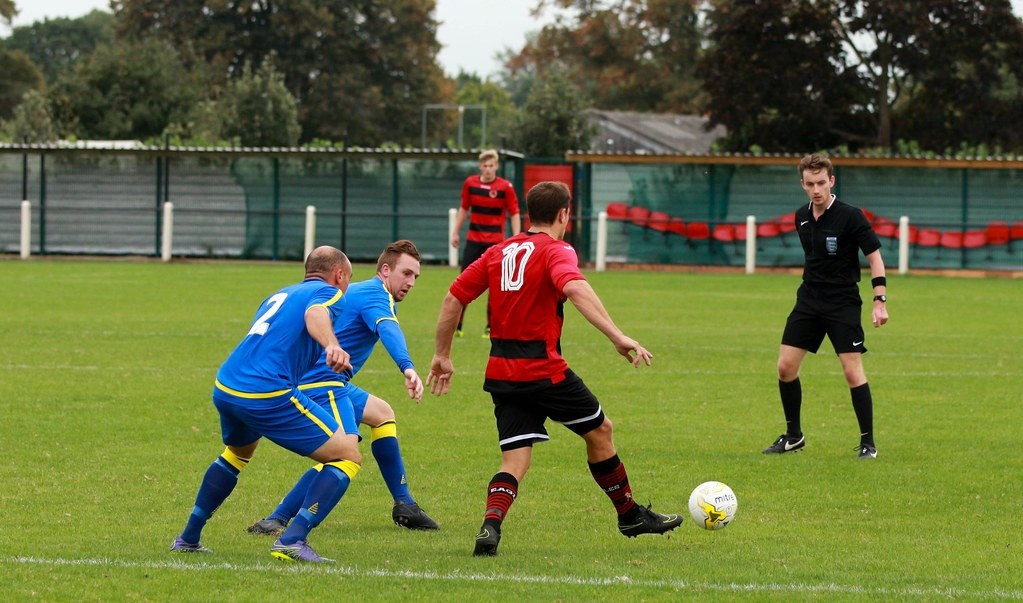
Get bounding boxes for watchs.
[874,295,886,302]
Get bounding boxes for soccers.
[688,480,738,531]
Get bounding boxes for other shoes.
[454,330,463,337]
[482,328,490,338]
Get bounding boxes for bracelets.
[872,276,886,288]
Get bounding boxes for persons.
[169,246,362,564]
[248,240,441,535]
[450,151,521,338]
[424,181,684,555]
[762,154,889,459]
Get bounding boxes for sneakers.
[271,537,337,564]
[763,432,806,454]
[392,502,438,529]
[618,503,684,538]
[472,524,501,554]
[248,518,286,535]
[854,444,877,457]
[170,537,210,552]
